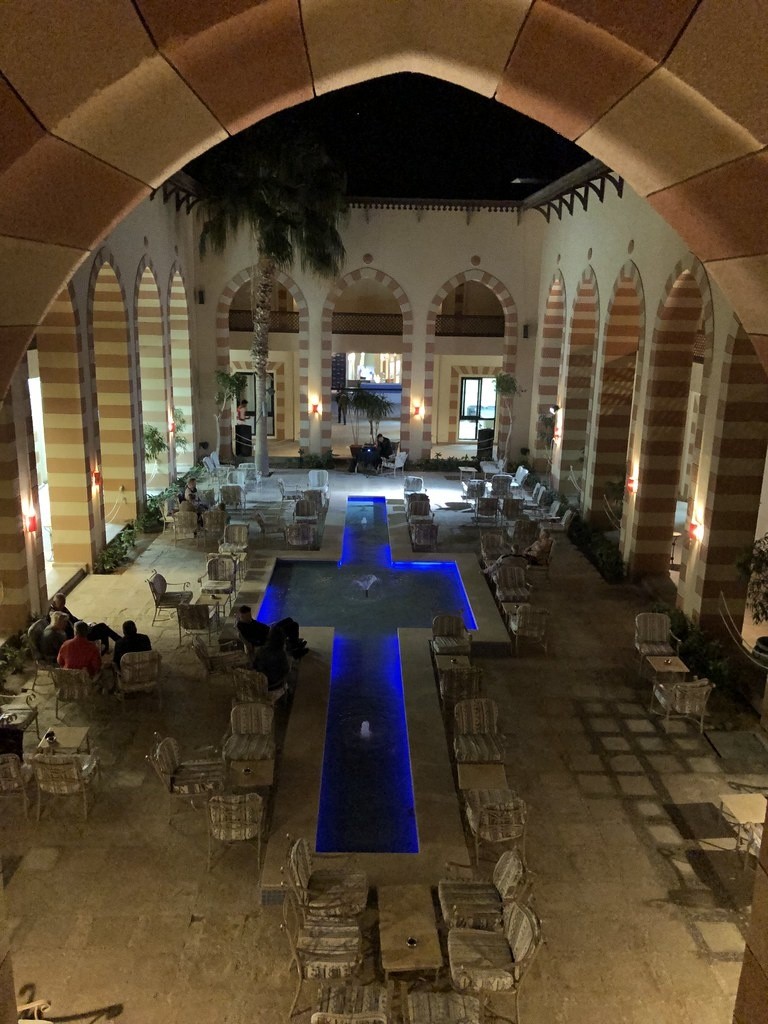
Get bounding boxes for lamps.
[549,405,560,414]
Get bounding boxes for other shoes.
[293,648,308,660]
[298,641,307,648]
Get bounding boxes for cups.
[451,658,456,663]
[664,658,671,663]
[243,768,251,774]
[406,937,418,947]
[515,605,519,608]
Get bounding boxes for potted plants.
[337,380,396,459]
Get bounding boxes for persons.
[39,611,105,662]
[238,399,248,421]
[180,478,231,536]
[336,390,349,425]
[374,434,392,470]
[56,621,101,678]
[108,620,151,695]
[479,527,552,575]
[47,593,122,648]
[236,605,311,684]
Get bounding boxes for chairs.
[0,451,768,1024]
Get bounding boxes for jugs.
[45,727,56,743]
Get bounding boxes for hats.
[74,621,89,635]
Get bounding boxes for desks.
[376,883,435,926]
[458,764,508,798]
[716,793,768,851]
[229,759,275,800]
[484,560,497,568]
[38,727,89,756]
[646,656,690,710]
[502,603,531,626]
[378,923,444,992]
[236,469,255,485]
[432,654,470,677]
[669,532,681,568]
[196,593,229,622]
[220,552,246,580]
[480,461,500,481]
